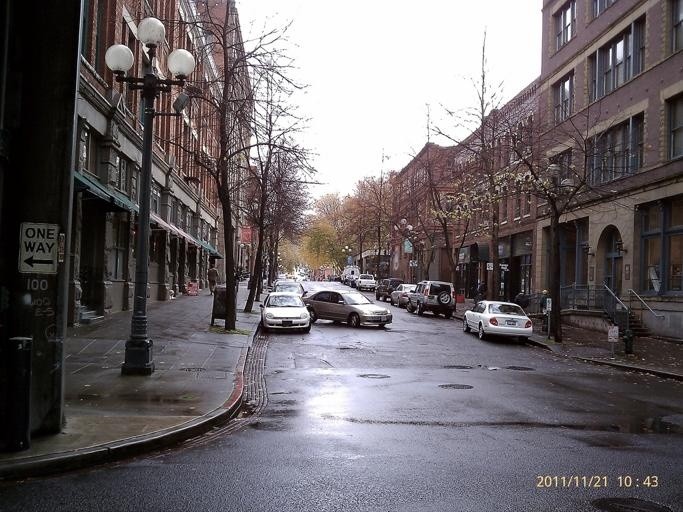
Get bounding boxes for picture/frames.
[589,267,594,281]
[625,264,630,280]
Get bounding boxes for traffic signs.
[18,223,58,275]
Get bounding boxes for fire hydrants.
[623,330,633,353]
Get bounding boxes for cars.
[260,265,533,343]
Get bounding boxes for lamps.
[580,240,594,257]
[523,235,533,250]
[615,238,627,256]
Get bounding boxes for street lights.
[393,219,416,283]
[529,163,575,340]
[105,17,195,375]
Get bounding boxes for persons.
[515,290,528,310]
[207,265,219,295]
[479,281,486,297]
[539,289,550,312]
